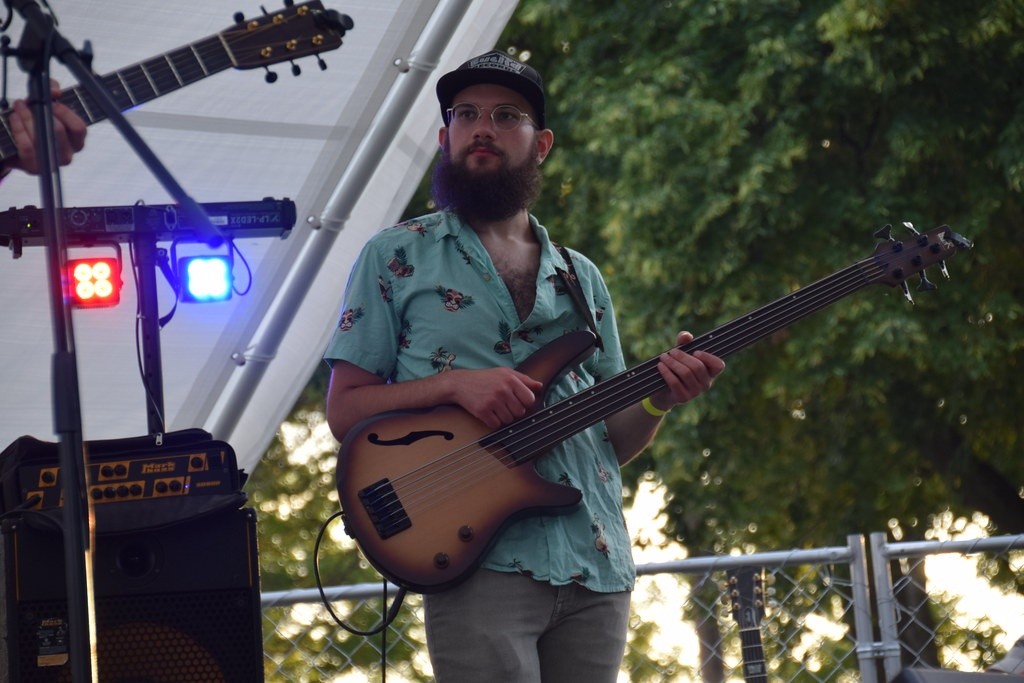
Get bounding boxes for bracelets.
[642,396,675,417]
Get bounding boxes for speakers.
[1,428,262,683]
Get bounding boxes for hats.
[436,51,545,128]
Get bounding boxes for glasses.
[447,102,541,131]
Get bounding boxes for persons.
[0,77,85,185]
[323,49,724,683]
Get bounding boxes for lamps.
[64,257,122,308]
[177,253,234,304]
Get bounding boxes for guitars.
[333,221,934,594]
[1,0,344,164]
